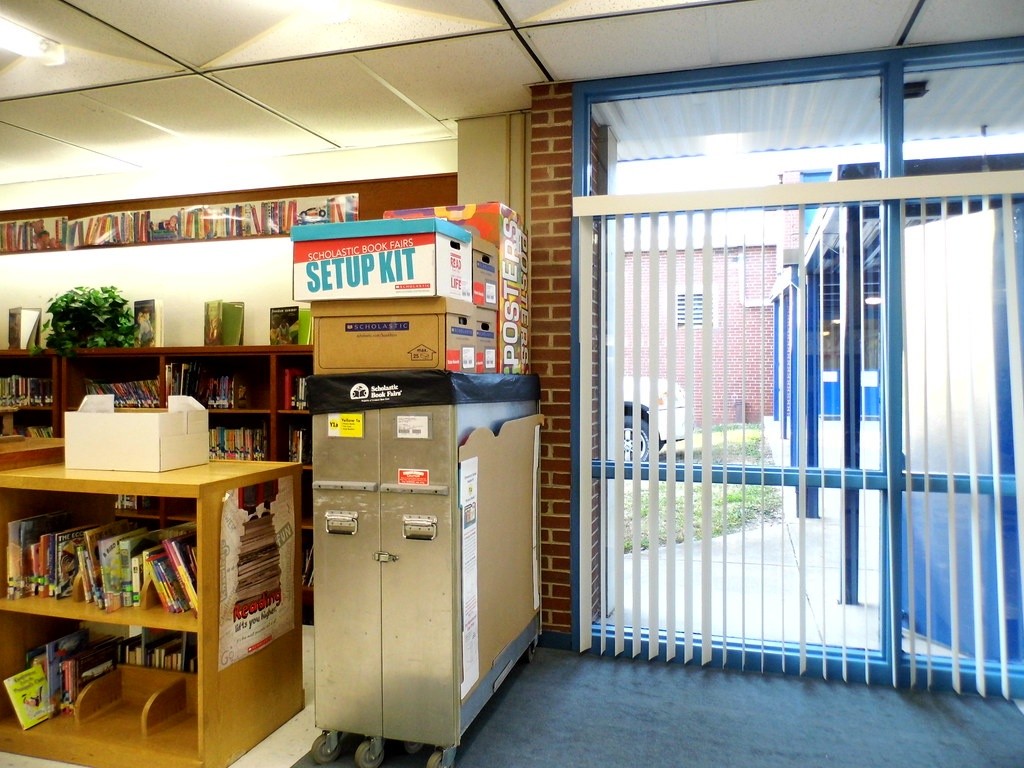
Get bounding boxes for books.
[288,424,312,463]
[9,307,42,351]
[166,364,248,409]
[13,427,53,438]
[134,299,162,347]
[0,374,53,406]
[1,628,197,731]
[209,421,268,461]
[86,377,159,408]
[205,301,242,346]
[270,306,313,346]
[283,368,306,409]
[2,511,198,619]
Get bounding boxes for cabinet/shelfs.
[0,346,314,768]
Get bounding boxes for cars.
[621,377,695,464]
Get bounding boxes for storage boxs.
[291,201,531,375]
[62,391,209,472]
